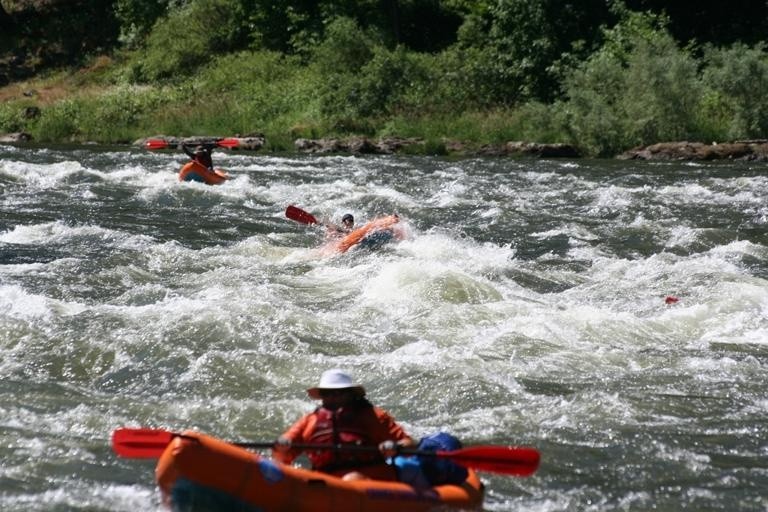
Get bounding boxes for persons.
[271,369,416,483]
[324,213,354,241]
[181,142,213,172]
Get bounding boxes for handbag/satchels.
[385,432,469,488]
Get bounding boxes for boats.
[156,430,485,512]
[178,161,227,186]
[308,214,400,262]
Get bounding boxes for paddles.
[285,205,354,235]
[146,139,239,149]
[112,429,540,476]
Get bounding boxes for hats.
[341,214,353,224]
[307,369,366,400]
